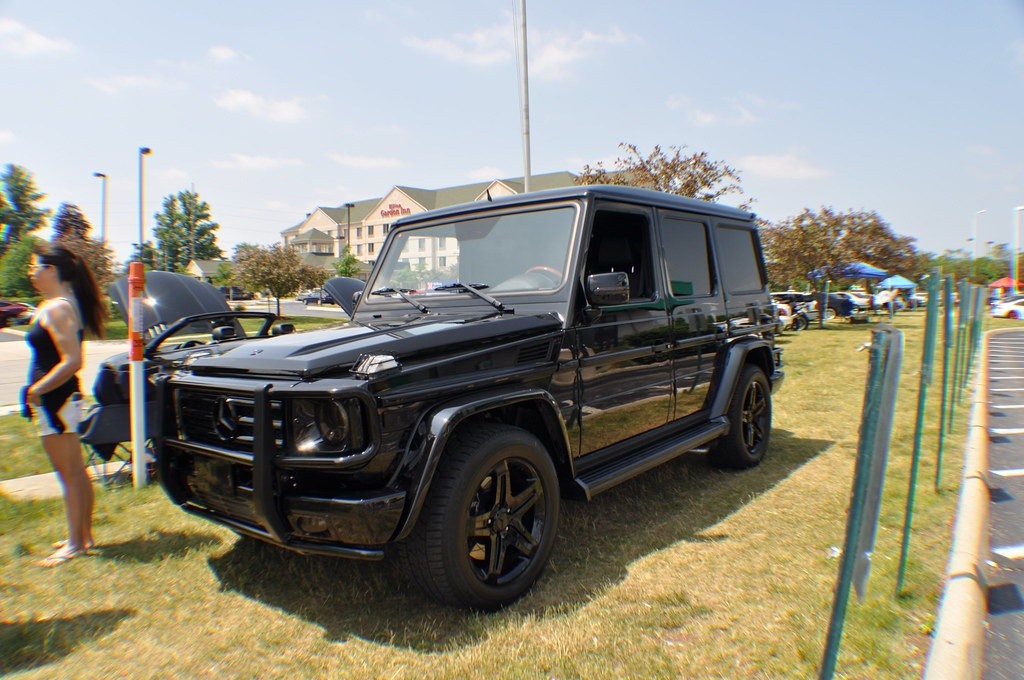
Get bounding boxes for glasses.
[24,261,54,272]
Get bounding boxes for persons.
[15,242,107,567]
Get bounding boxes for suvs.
[141,183,788,615]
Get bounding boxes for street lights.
[91,172,107,249]
[136,147,154,247]
[972,208,990,282]
[344,202,355,256]
[1013,205,1024,293]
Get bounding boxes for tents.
[808,261,893,322]
[873,275,918,312]
[988,277,1024,288]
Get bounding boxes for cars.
[988,294,1024,321]
[217,286,255,300]
[90,268,368,409]
[0,302,37,325]
[302,291,336,307]
[767,286,928,330]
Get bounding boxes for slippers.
[52,539,94,552]
[41,550,86,568]
